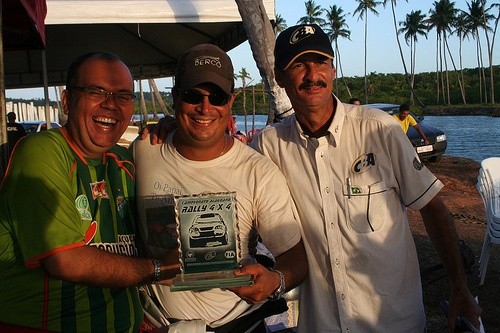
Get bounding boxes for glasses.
[65,86,137,106]
[176,88,230,106]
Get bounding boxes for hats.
[8,112,15,119]
[273,23,334,72]
[174,43,234,96]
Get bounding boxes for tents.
[0,1,277,129]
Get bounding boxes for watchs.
[269,266,286,301]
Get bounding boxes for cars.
[358,103,447,164]
[187,211,228,248]
[131,114,142,127]
[17,121,63,136]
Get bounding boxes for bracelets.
[151,257,161,281]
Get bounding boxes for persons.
[351,98,361,106]
[5,112,27,154]
[392,102,430,148]
[0,24,483,333]
